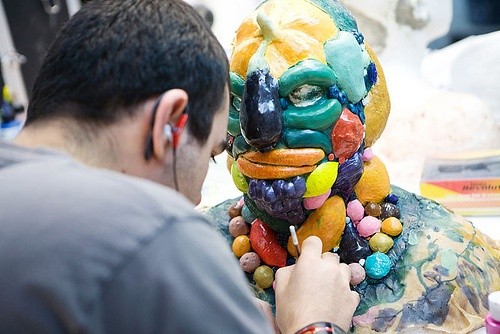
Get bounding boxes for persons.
[199,0,500,334]
[0,1,360,334]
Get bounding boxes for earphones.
[144,92,190,160]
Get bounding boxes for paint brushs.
[290,226,301,265]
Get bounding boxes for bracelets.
[295,322,347,334]
